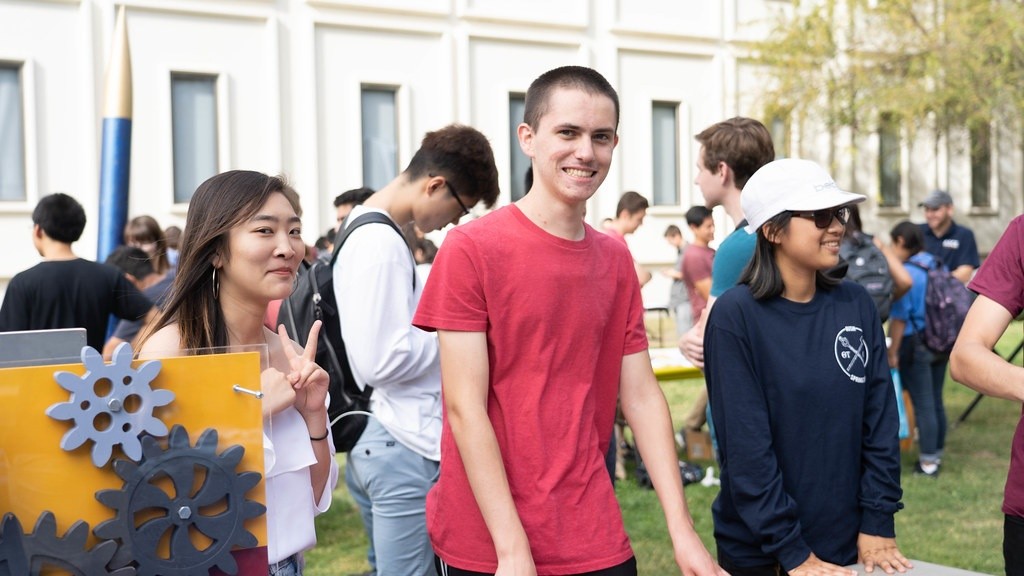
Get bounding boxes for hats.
[741,158,867,235]
[917,191,953,210]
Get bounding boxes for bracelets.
[310,427,329,441]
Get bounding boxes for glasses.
[785,207,852,229]
[430,173,470,218]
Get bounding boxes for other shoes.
[912,460,940,476]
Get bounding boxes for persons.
[0,194,162,360]
[682,207,720,326]
[138,168,340,576]
[525,164,587,224]
[912,189,982,285]
[695,115,776,457]
[886,220,950,478]
[703,158,918,576]
[410,64,731,576]
[836,202,913,327]
[599,216,614,230]
[597,189,654,493]
[312,227,337,251]
[950,214,1024,576]
[331,122,500,576]
[334,181,376,228]
[660,225,694,343]
[102,214,181,362]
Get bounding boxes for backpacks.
[843,231,894,325]
[903,258,972,357]
[274,213,416,453]
